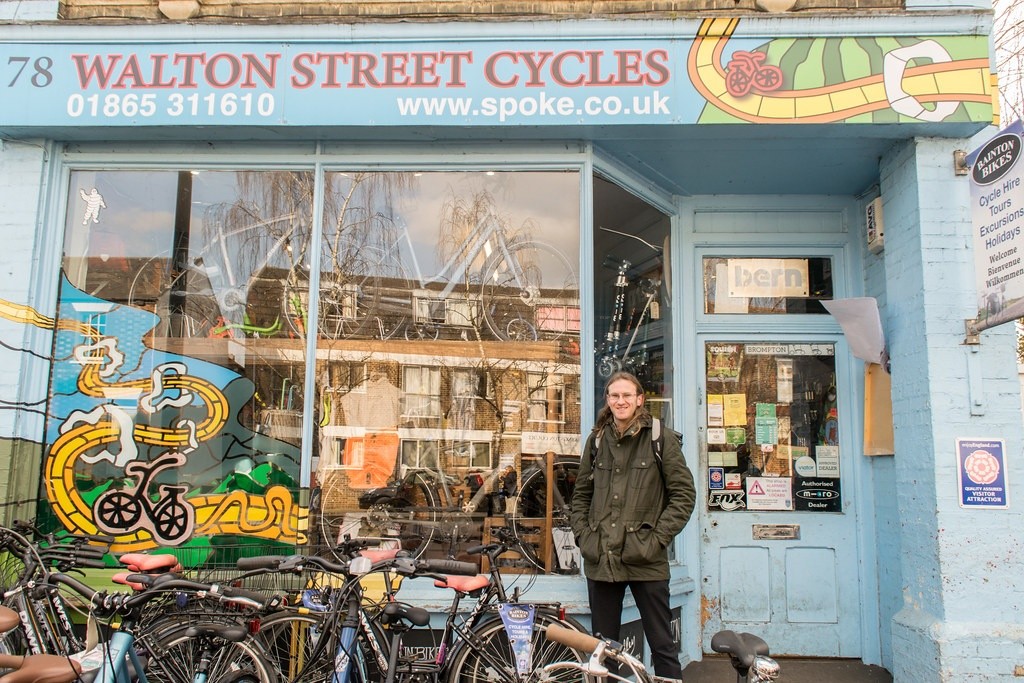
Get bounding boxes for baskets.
[172,545,344,606]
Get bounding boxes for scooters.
[594,254,662,383]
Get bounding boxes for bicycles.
[321,394,580,576]
[307,173,578,344]
[0,516,780,683]
[128,197,381,340]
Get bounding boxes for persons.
[570,373,696,683]
[730,444,763,484]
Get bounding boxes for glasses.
[606,393,638,400]
